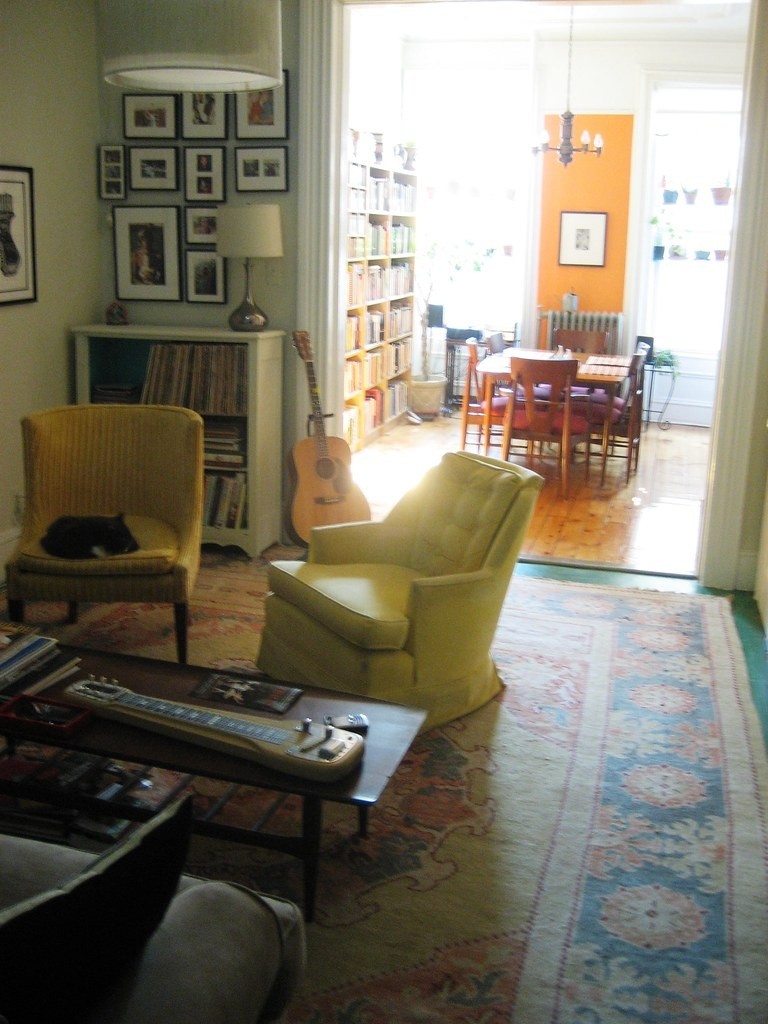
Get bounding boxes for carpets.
[1,548,767,1024]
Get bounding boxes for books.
[388,338,411,375]
[345,354,384,392]
[0,620,84,696]
[92,381,136,403]
[204,419,247,468]
[348,216,415,259]
[348,121,416,213]
[388,379,408,418]
[344,388,382,444]
[347,306,413,352]
[346,263,412,308]
[139,343,247,415]
[204,471,248,529]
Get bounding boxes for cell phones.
[331,713,369,730]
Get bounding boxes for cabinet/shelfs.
[69,322,284,558]
[340,122,415,455]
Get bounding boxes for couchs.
[9,405,204,667]
[0,835,308,1024]
[251,452,544,740]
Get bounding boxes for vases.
[406,372,450,418]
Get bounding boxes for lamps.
[215,200,282,330]
[95,0,282,101]
[530,1,603,167]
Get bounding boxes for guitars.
[280,329,376,546]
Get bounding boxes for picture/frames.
[0,164,37,307]
[97,69,290,305]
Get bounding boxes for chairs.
[462,330,653,484]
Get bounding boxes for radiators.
[537,309,624,355]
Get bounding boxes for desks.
[1,641,429,920]
[477,346,632,476]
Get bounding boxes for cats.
[40,512,141,561]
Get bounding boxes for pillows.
[0,773,203,1024]
